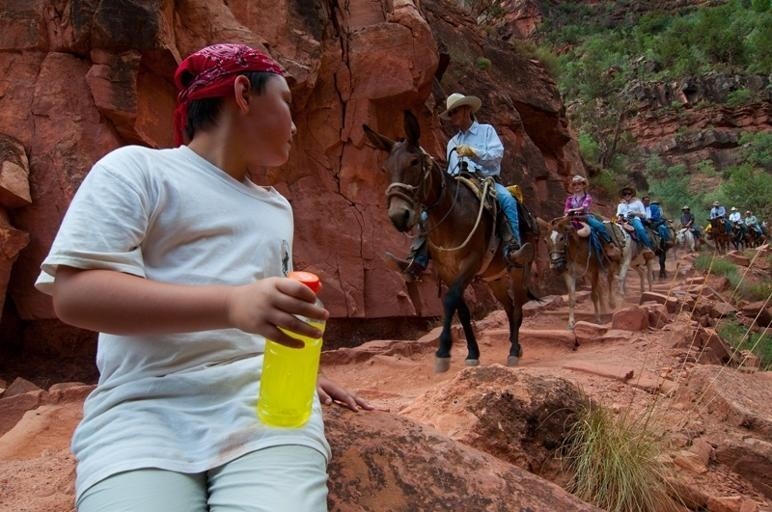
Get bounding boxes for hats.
[731,207,736,211]
[651,200,660,205]
[438,92,483,121]
[618,185,636,199]
[569,175,589,187]
[713,201,720,206]
[682,205,690,211]
[747,211,751,214]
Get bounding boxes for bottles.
[257,270,329,433]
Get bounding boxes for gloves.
[456,144,475,156]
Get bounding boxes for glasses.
[447,109,458,117]
[622,192,632,196]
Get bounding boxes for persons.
[561,175,619,252]
[32,43,391,511]
[614,186,664,258]
[708,200,735,236]
[728,205,748,233]
[649,201,664,217]
[398,90,535,281]
[680,204,701,240]
[637,196,671,242]
[743,211,762,240]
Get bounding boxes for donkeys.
[362,108,539,373]
[615,217,769,295]
[537,217,625,330]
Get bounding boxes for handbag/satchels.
[512,196,533,232]
[666,227,677,245]
[603,220,626,247]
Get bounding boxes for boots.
[501,240,534,269]
[382,252,430,284]
